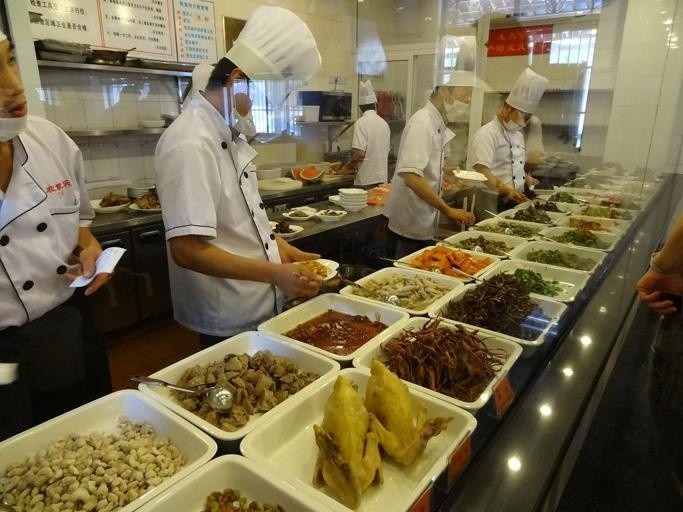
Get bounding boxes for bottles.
[375,91,403,118]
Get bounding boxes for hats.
[357,80,377,106]
[433,35,477,88]
[505,68,549,115]
[223,5,322,111]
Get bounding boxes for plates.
[266,204,348,237]
[138,120,166,128]
[84,198,129,214]
[326,188,368,212]
[287,255,339,283]
[129,201,162,216]
[451,168,489,184]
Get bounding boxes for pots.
[89,47,135,64]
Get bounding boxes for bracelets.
[649,253,676,274]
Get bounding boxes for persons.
[156,33,322,345]
[634,210,682,316]
[381,85,474,263]
[0,30,113,432]
[341,101,391,189]
[466,98,539,225]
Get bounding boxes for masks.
[502,114,523,134]
[0,114,28,143]
[443,95,469,123]
[229,76,265,138]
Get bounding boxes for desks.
[266,182,482,268]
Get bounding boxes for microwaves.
[299,91,351,120]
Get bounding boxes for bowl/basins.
[123,185,156,201]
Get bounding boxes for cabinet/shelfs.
[83,221,169,336]
[266,187,338,213]
[36,57,192,207]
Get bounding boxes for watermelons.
[290,166,325,183]
